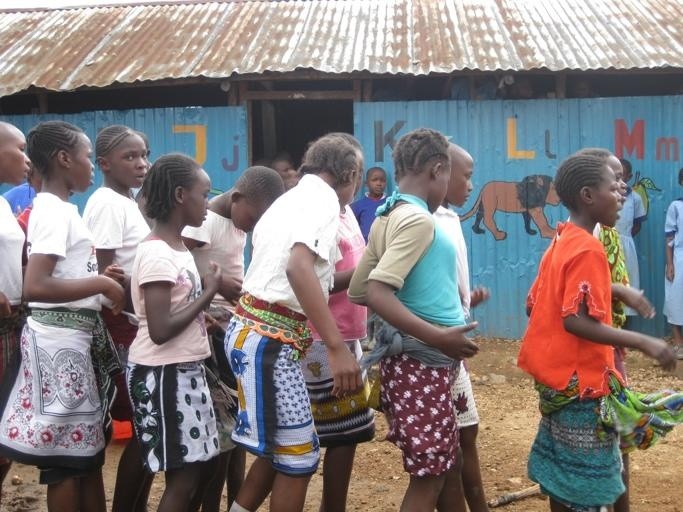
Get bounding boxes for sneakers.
[671,343,683,360]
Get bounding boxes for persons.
[0,119,491,512]
[519,149,682,511]
